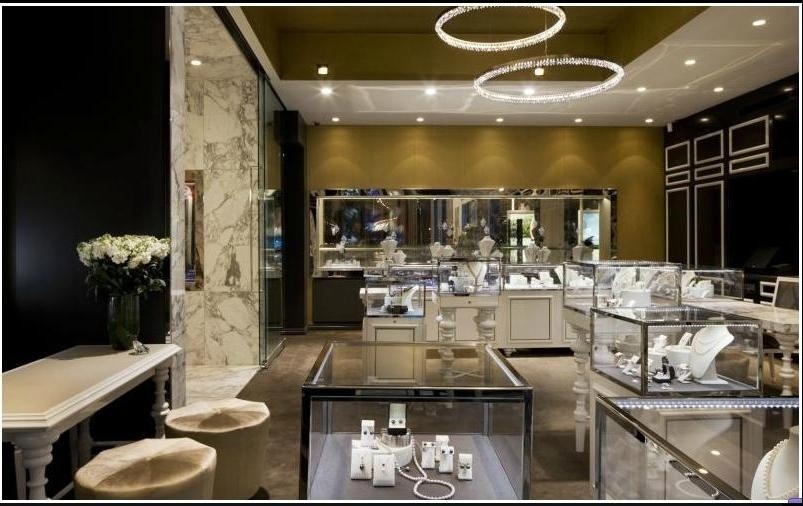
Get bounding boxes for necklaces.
[376,437,455,500]
[692,328,729,354]
[762,438,799,500]
[466,262,483,286]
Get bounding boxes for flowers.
[75,230,171,348]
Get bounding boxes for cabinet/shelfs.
[681,266,744,300]
[300,339,532,499]
[587,306,768,500]
[561,262,681,454]
[434,257,503,408]
[594,394,798,499]
[424,265,613,356]
[362,264,425,387]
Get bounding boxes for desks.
[686,294,796,397]
[2,342,185,499]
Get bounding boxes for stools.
[165,395,270,499]
[73,435,218,499]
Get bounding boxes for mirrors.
[308,187,619,264]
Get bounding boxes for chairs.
[752,274,799,383]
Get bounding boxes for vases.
[105,293,141,350]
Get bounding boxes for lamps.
[471,4,626,108]
[436,5,566,53]
